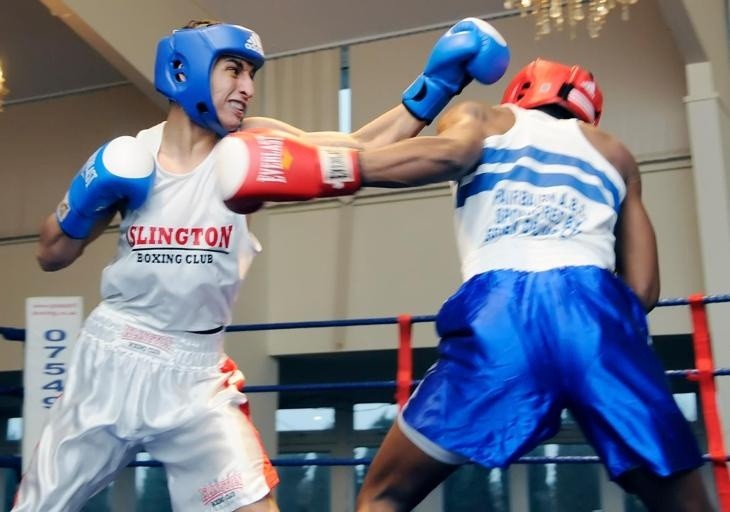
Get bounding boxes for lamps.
[501,0,637,41]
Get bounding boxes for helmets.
[497,53,605,131]
[150,23,267,139]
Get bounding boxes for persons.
[10,17,511,512]
[217,59,714,512]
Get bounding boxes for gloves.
[53,131,157,243]
[401,16,510,129]
[209,119,364,217]
